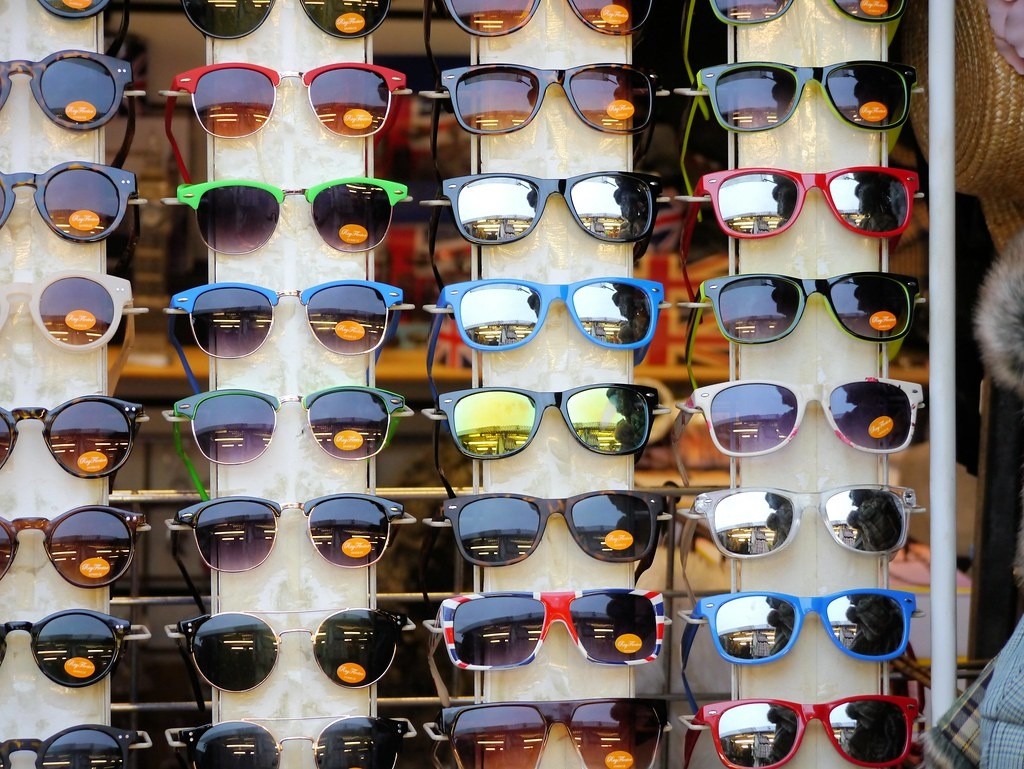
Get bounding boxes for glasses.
[426,170,663,320]
[683,694,924,769]
[173,716,409,769]
[682,0,908,120]
[679,60,917,223]
[432,382,660,502]
[0,270,135,397]
[176,606,408,716]
[678,167,921,325]
[429,62,658,187]
[419,489,665,607]
[679,486,917,609]
[0,724,138,769]
[426,277,666,432]
[0,504,147,589]
[181,0,392,40]
[0,49,135,169]
[678,587,917,715]
[432,698,669,769]
[0,395,147,495]
[175,176,408,256]
[684,270,923,390]
[670,377,926,487]
[171,491,406,614]
[425,588,666,707]
[38,0,130,57]
[165,280,404,395]
[421,0,654,111]
[0,161,140,244]
[0,609,131,688]
[164,61,407,186]
[171,384,406,502]
